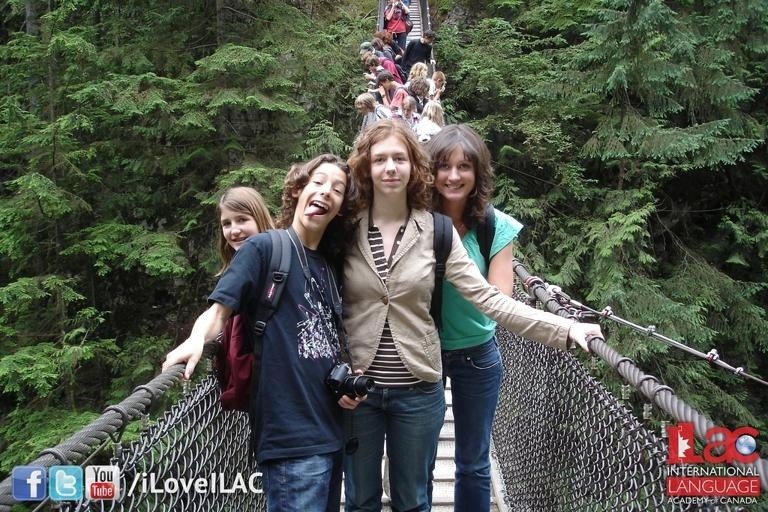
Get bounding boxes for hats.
[374,70,394,89]
[359,41,372,55]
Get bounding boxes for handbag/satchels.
[401,7,413,34]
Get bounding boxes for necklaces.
[457,221,467,232]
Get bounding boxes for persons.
[354,0,447,148]
[335,119,607,511]
[162,153,375,511]
[212,185,280,279]
[427,124,523,510]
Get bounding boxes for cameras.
[325,362,376,400]
[394,2,402,6]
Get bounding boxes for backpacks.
[210,229,292,413]
